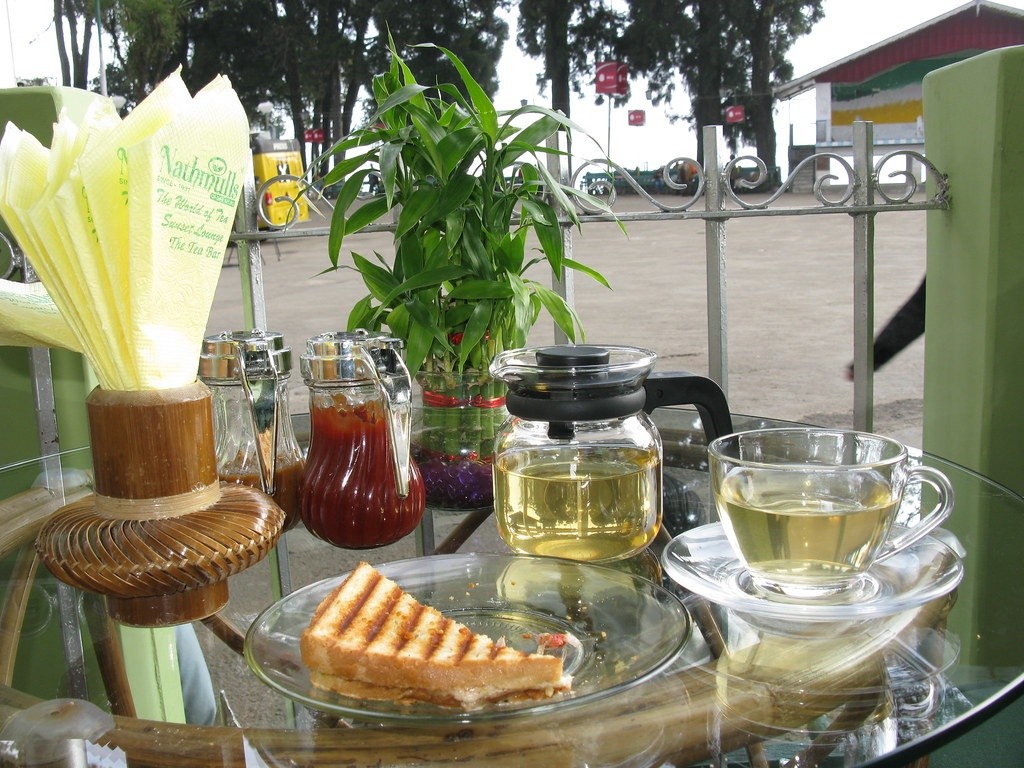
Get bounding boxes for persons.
[844,270,925,383]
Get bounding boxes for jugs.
[485,336,738,563]
[300,325,425,550]
[202,332,305,535]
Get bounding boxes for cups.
[705,425,954,596]
[700,676,943,768]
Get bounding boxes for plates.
[658,521,966,620]
[245,550,694,728]
[662,588,965,704]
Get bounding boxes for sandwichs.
[296,560,571,710]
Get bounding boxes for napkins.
[1,70,251,390]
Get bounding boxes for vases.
[286,18,636,513]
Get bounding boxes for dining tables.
[1,408,1022,766]
